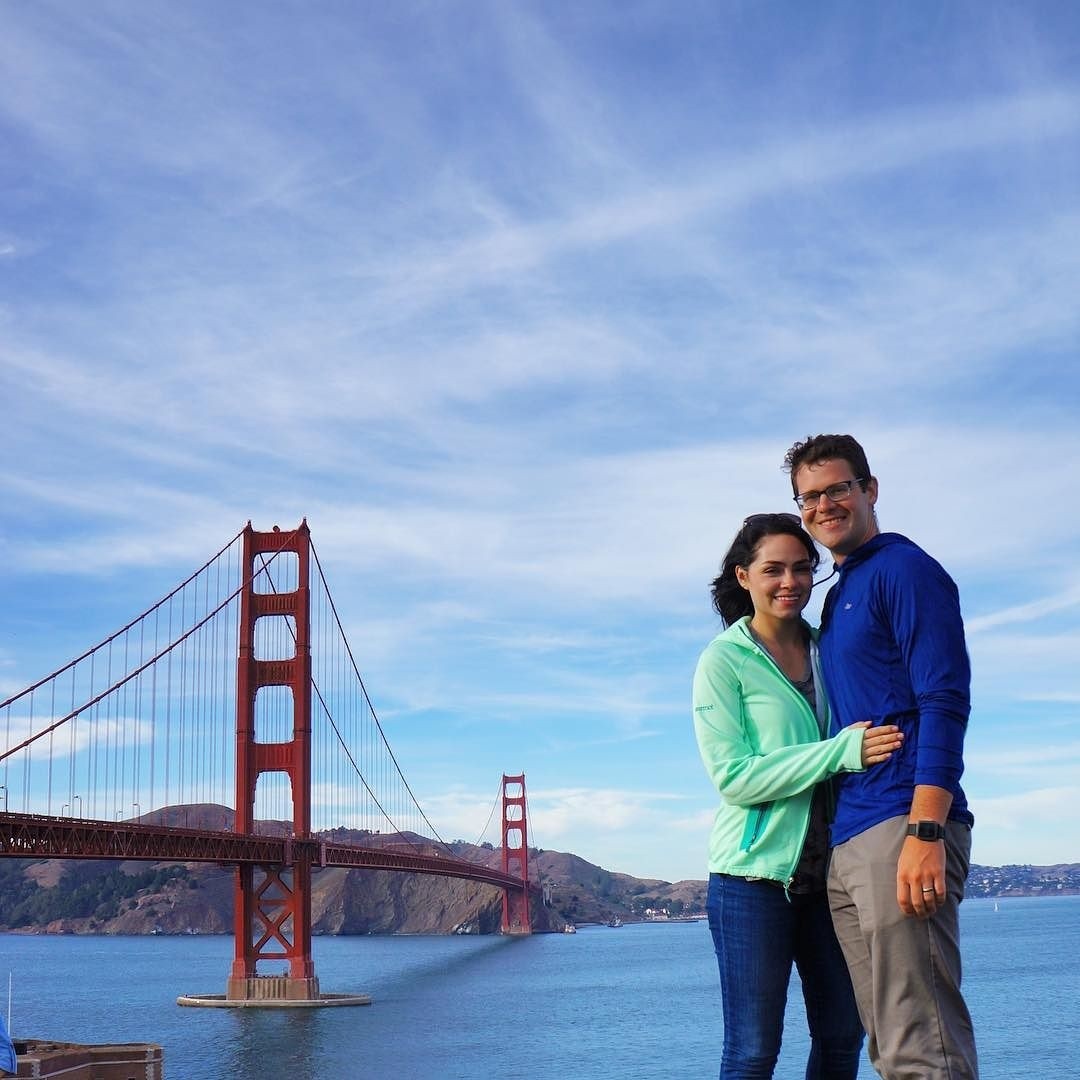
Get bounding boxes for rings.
[922,887,934,892]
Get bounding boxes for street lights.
[62,804,69,816]
[0,785,9,812]
[116,810,122,822]
[133,803,141,824]
[74,795,82,819]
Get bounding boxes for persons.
[780,430,983,1080]
[691,511,904,1080]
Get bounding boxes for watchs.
[906,820,945,841]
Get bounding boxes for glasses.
[793,477,867,512]
[743,514,802,541]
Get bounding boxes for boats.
[607,912,624,928]
